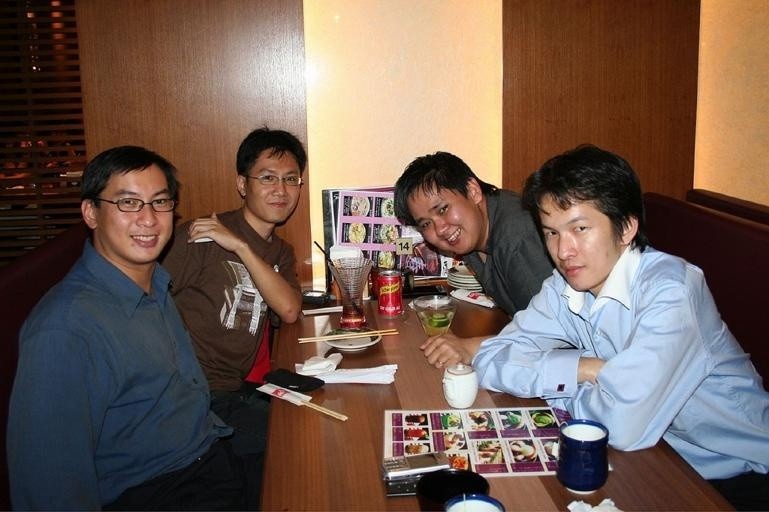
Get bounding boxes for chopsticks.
[297,328,399,345]
[255,382,348,422]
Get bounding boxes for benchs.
[643,191,769,393]
[0,220,90,512]
[687,188,769,225]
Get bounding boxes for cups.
[442,493,505,512]
[327,257,373,331]
[555,418,610,495]
[412,295,457,337]
[414,468,490,512]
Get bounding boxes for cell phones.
[263,367,326,394]
[385,479,429,497]
[384,452,452,479]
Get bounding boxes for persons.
[393,152,555,370]
[8,145,262,512]
[471,142,768,511]
[157,127,307,474]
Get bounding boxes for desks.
[259,298,737,512]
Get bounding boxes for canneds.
[377,271,404,319]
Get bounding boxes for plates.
[322,327,382,351]
[446,266,483,293]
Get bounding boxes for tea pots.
[441,362,478,409]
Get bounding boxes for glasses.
[247,174,303,186]
[97,197,176,213]
